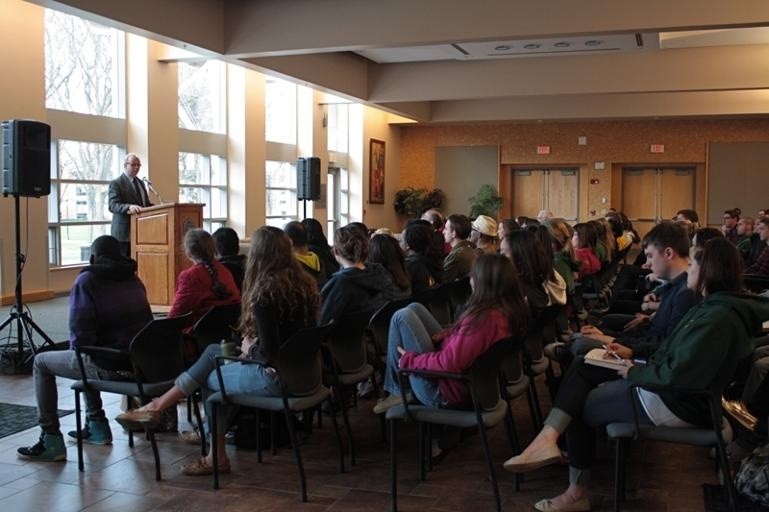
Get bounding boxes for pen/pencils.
[602,344,621,360]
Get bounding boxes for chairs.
[69,312,206,482]
[491,328,541,483]
[210,318,345,503]
[316,307,388,433]
[418,274,471,324]
[366,295,429,401]
[385,343,518,494]
[185,301,243,423]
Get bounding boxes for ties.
[132,179,144,207]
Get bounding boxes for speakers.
[297,158,320,201]
[0,119,50,198]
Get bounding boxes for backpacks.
[734,442,769,502]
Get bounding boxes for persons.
[107,155,154,257]
[115,208,768,511]
[17,234,153,462]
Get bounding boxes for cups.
[220,339,238,363]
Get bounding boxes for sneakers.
[372,393,413,415]
[179,457,231,476]
[115,410,161,428]
[67,418,113,446]
[16,433,68,462]
[721,395,758,431]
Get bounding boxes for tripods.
[0,194,57,368]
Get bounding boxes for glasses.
[128,162,141,166]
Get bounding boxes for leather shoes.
[534,495,590,512]
[502,446,563,474]
[180,430,206,444]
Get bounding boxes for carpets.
[1,402,79,439]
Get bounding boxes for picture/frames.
[368,139,386,204]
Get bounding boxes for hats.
[470,214,498,237]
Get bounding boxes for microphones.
[142,176,164,205]
